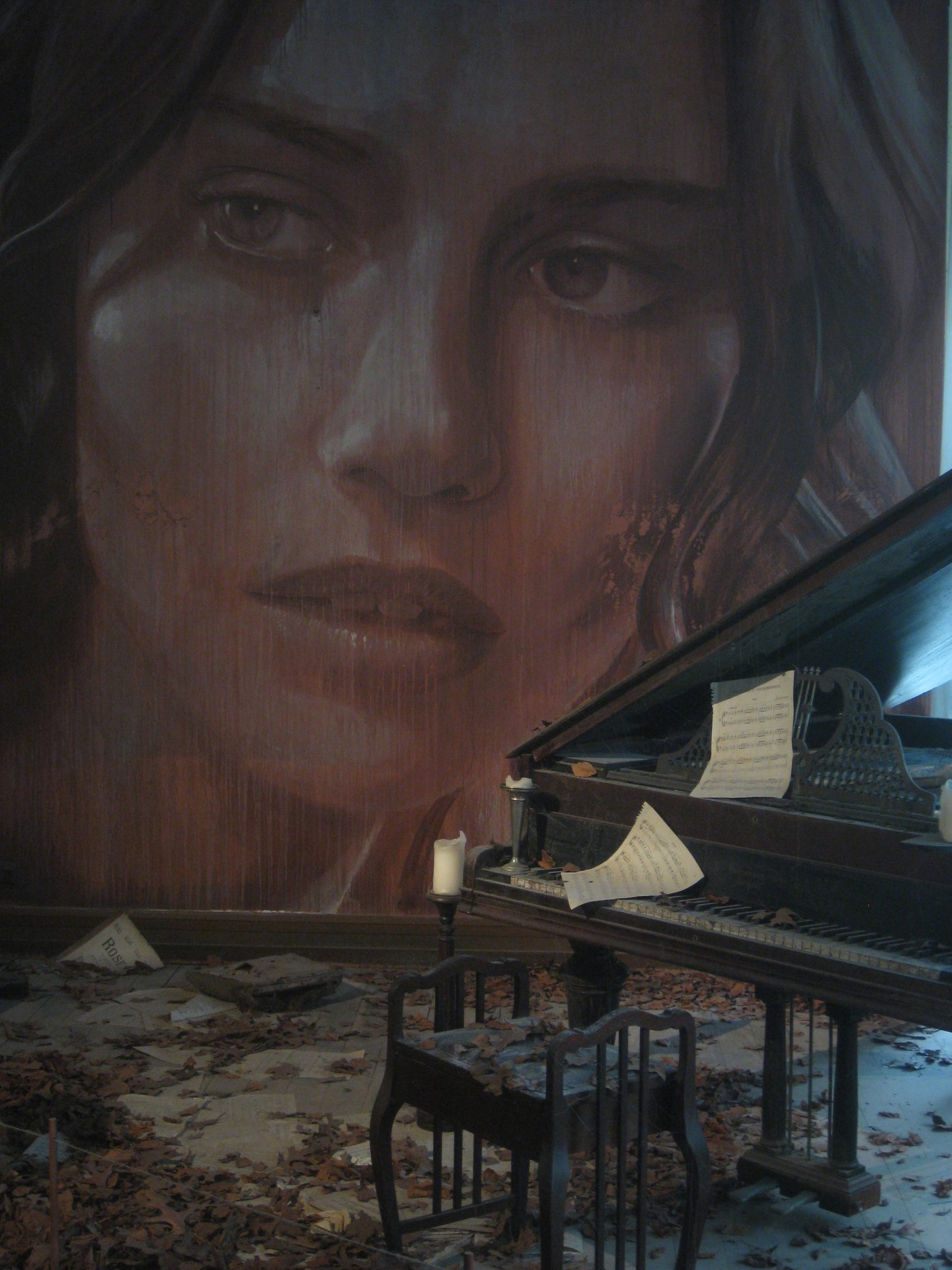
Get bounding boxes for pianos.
[457,467,952,1219]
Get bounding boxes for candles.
[433,829,467,895]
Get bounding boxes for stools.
[369,953,710,1269]
